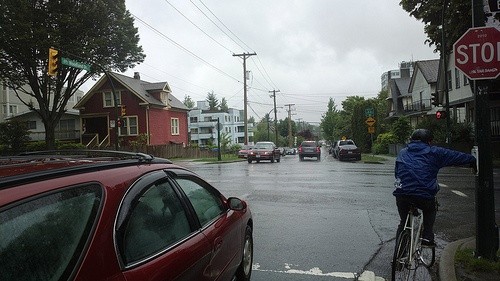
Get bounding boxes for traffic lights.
[49,48,59,76]
[430,92,439,107]
[121,105,126,117]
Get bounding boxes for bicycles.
[391,204,436,281]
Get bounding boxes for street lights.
[267,107,284,141]
[210,116,221,161]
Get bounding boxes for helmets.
[411,129,434,142]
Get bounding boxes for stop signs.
[453,27,500,81]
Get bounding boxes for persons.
[392,129,477,271]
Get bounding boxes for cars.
[0,150,254,281]
[278,146,299,157]
[238,145,254,159]
[338,145,361,161]
[247,142,281,163]
[328,140,355,159]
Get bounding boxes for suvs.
[298,140,322,161]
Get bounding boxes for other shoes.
[422,239,438,248]
